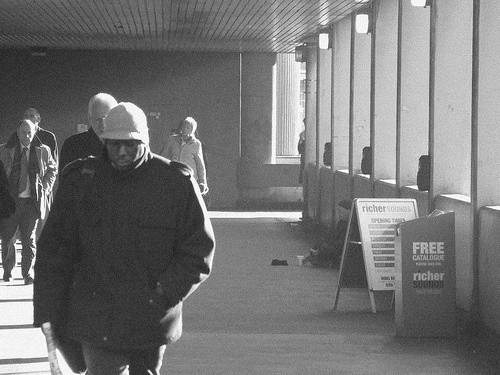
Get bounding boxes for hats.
[101,102,150,145]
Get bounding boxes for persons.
[21,107,59,246]
[32,101,218,375]
[296,118,308,185]
[161,114,210,198]
[59,93,120,192]
[0,118,58,285]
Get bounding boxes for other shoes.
[3,274,11,283]
[24,274,33,285]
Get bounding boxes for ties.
[18,147,28,193]
